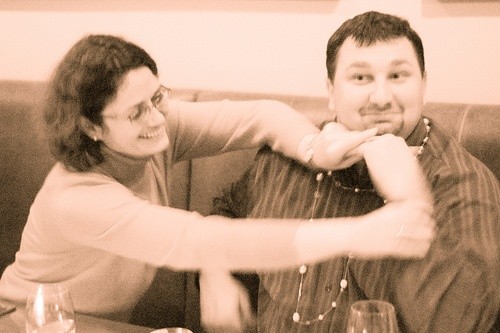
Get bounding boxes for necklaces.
[293,115,432,324]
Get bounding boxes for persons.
[192,11,500,333]
[0,33,433,332]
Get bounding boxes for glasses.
[98,86,171,126]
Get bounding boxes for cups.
[347,300,399,333]
[24,283,76,333]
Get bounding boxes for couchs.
[0,79,500,333]
